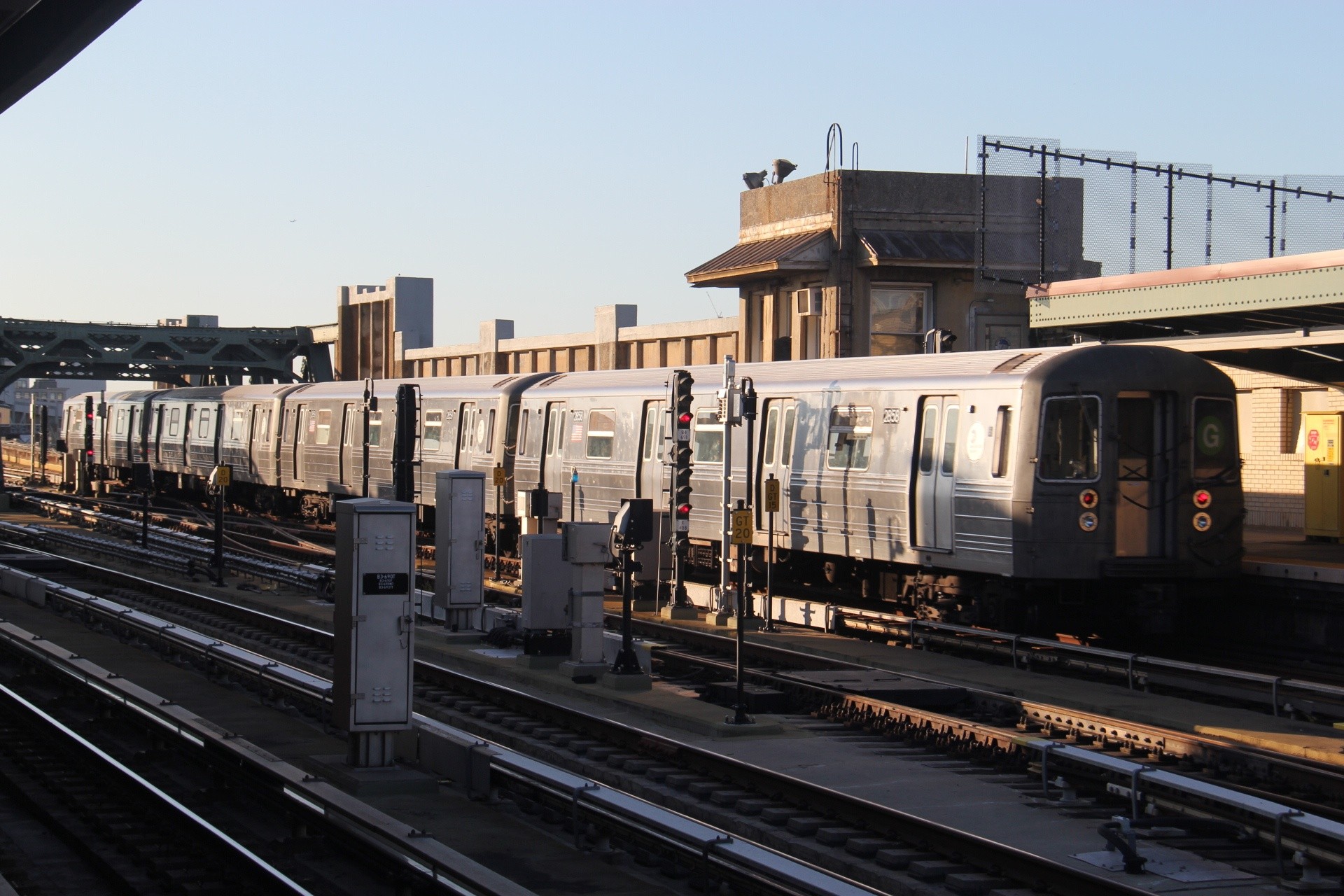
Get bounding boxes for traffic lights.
[936,330,958,354]
[84,396,94,462]
[672,371,694,533]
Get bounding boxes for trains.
[59,343,1248,649]
